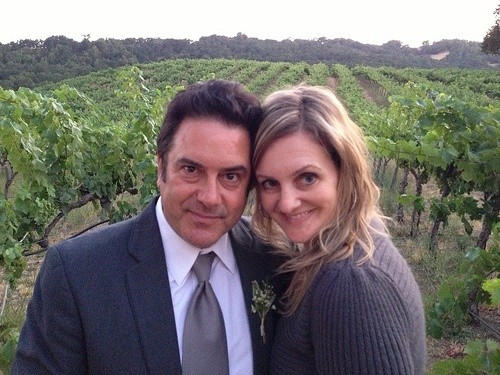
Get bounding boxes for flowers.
[251,280,276,345]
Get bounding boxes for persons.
[10,78,300,375]
[252,86,426,375]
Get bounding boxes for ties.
[182,252,230,375]
